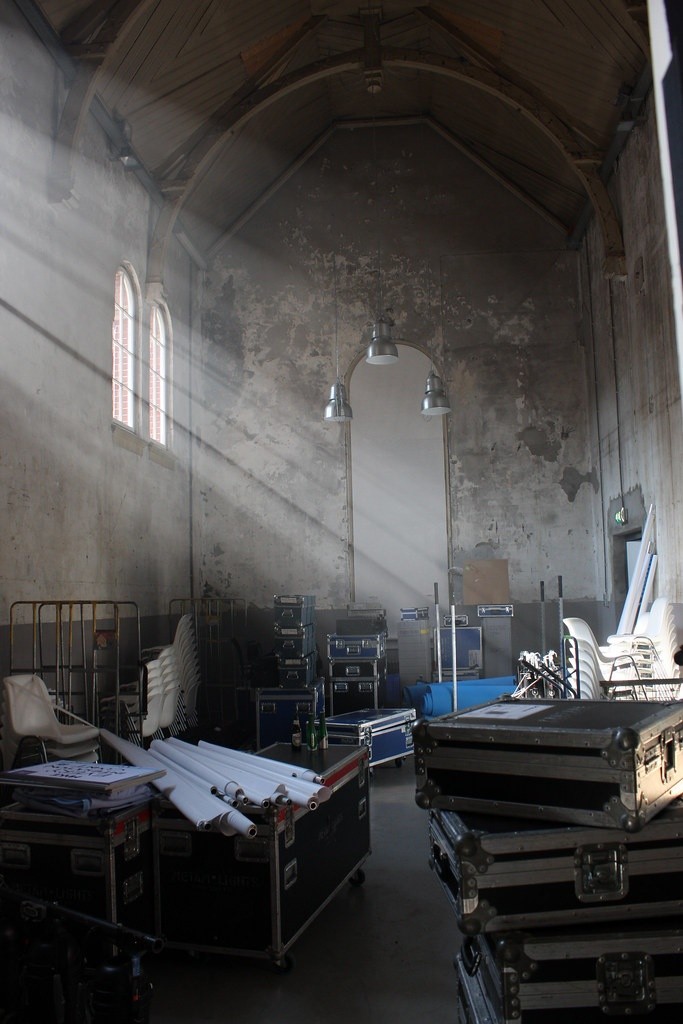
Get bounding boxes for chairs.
[0,613,201,772]
[563,598,683,701]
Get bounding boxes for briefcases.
[477,605,514,617]
[411,695,683,1024]
[444,615,468,627]
[346,602,386,620]
[337,618,388,637]
[329,656,388,678]
[400,607,430,622]
[327,634,386,660]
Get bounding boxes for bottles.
[292,716,302,751]
[319,711,328,749]
[307,713,317,751]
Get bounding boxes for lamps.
[364,248,398,365]
[323,254,354,423]
[419,264,452,415]
[116,147,142,172]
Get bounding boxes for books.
[0,760,167,794]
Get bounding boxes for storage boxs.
[462,911,683,1024]
[426,795,683,935]
[0,800,156,936]
[412,693,683,834]
[236,595,514,782]
[453,951,501,1024]
[151,741,372,974]
